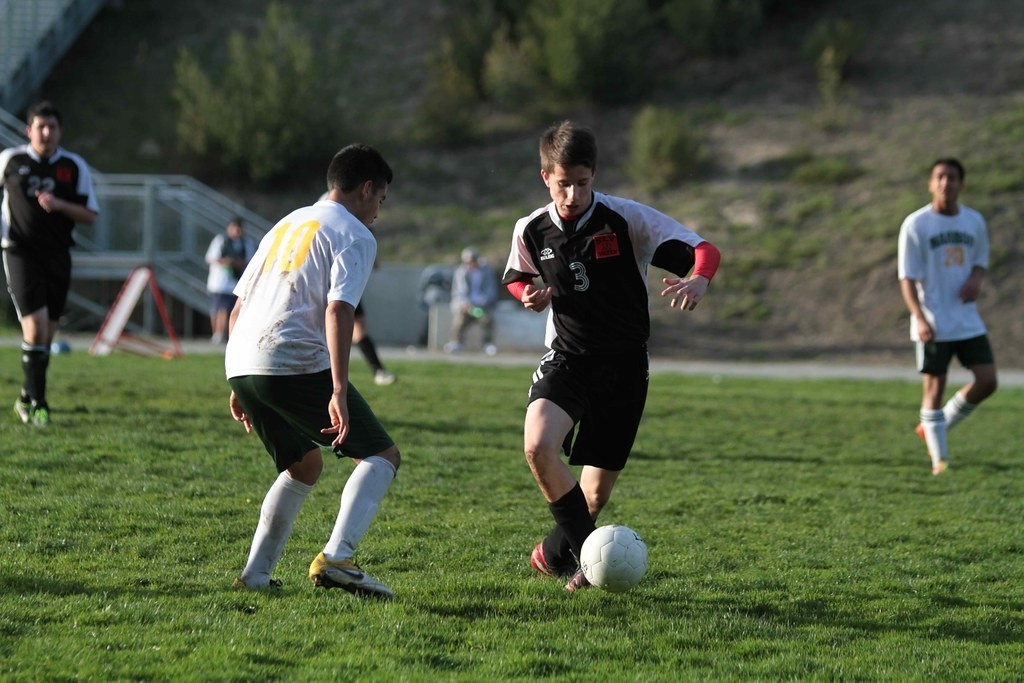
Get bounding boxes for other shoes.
[916,424,927,441]
[933,461,951,476]
[374,371,396,386]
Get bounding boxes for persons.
[0,102,100,430]
[205,144,401,599]
[898,158,998,476]
[503,121,720,592]
[444,246,500,357]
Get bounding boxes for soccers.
[579,524,649,594]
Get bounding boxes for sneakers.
[14,397,31,423]
[531,542,580,578]
[232,577,282,592]
[30,400,50,427]
[565,568,590,592]
[309,551,394,602]
